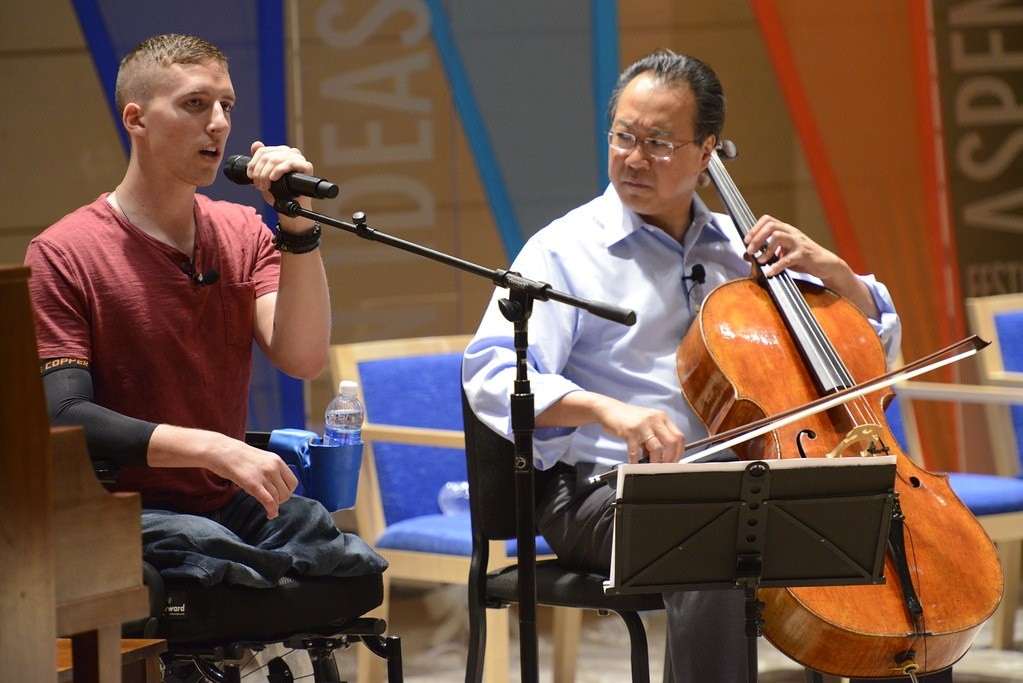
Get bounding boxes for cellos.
[676,138,1005,683]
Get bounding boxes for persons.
[23,31,391,586]
[462,47,901,683]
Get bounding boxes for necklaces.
[112,185,134,226]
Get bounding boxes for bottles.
[323,381,365,447]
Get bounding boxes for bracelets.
[269,215,320,253]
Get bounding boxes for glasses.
[604,129,700,158]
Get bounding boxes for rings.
[644,435,656,443]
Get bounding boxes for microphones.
[692,265,705,284]
[224,155,339,200]
[197,270,222,287]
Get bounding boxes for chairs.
[964,289,1023,480]
[457,362,665,683]
[332,337,583,683]
[882,346,1023,650]
